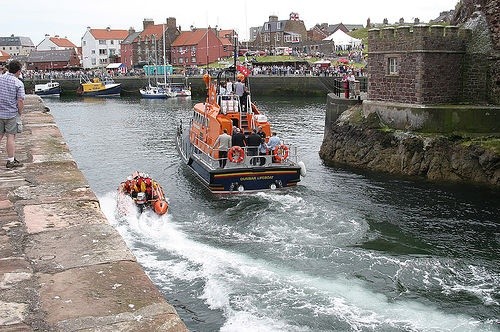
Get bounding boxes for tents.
[322,29,362,51]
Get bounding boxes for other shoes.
[6,158,24,167]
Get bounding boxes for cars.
[238,46,290,57]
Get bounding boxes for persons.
[0,60,25,168]
[342,71,357,99]
[213,129,232,168]
[234,79,249,112]
[343,65,363,76]
[253,66,341,77]
[268,132,280,162]
[257,126,265,138]
[126,177,151,198]
[29,69,121,79]
[246,129,264,165]
[259,138,267,166]
[226,78,234,94]
[231,126,246,150]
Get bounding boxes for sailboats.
[139,53,192,99]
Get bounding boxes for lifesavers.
[227,146,245,162]
[274,144,289,161]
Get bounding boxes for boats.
[175,65,307,202]
[116,170,169,229]
[30,82,61,99]
[76,71,122,97]
